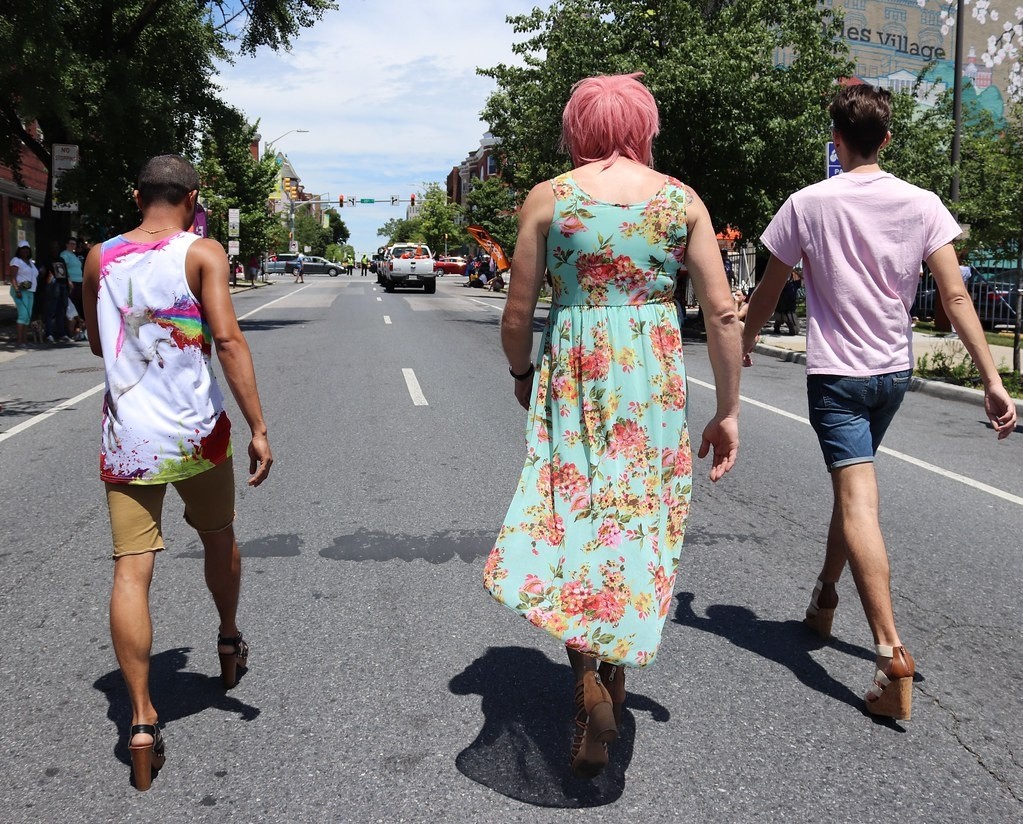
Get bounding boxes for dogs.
[30,319,46,344]
[71,315,89,341]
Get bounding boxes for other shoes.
[45,335,57,345]
[15,342,39,349]
[60,335,74,344]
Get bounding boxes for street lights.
[264,129,309,282]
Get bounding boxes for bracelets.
[509,361,534,380]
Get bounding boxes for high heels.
[797,575,840,649]
[866,644,914,721]
[217,630,249,688]
[566,662,627,779]
[126,723,166,791]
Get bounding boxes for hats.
[17,240,31,248]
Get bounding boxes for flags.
[466,225,511,272]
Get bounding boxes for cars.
[262,253,301,274]
[284,256,347,277]
[434,256,469,276]
[374,242,438,294]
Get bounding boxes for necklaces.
[138,226,180,234]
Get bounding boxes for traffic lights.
[411,194,415,206]
[340,195,344,208]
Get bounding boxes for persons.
[82,154,273,791]
[742,84,1017,720]
[294,252,304,283]
[360,253,368,276]
[733,290,759,342]
[9,237,95,348]
[344,255,355,275]
[247,253,260,289]
[483,75,743,781]
[465,256,505,292]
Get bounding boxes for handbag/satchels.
[20,280,33,290]
